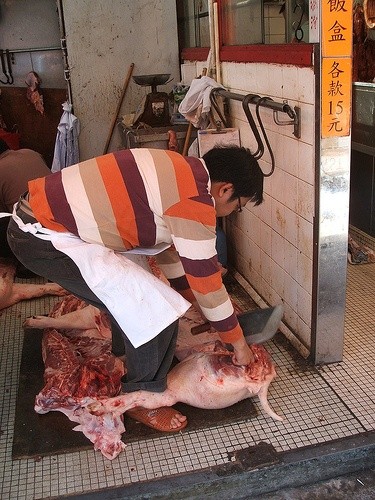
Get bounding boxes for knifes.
[191,304,285,352]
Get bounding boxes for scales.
[132,73,175,130]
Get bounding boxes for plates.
[131,73,172,86]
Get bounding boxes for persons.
[7,143,265,432]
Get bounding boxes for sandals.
[124,406,188,432]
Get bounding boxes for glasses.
[236,196,244,213]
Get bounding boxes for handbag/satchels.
[51,102,79,173]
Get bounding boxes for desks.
[117,120,200,157]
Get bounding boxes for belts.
[18,194,36,218]
